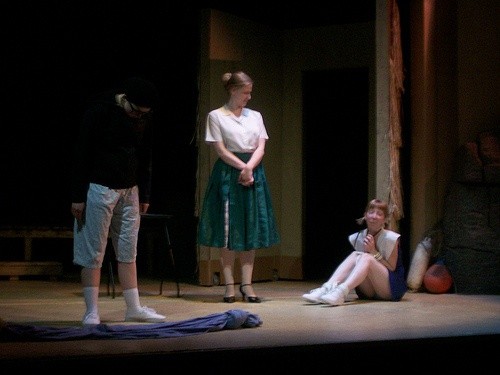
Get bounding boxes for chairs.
[107,190,180,297]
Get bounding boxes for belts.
[96,182,137,189]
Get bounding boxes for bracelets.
[374,252,383,261]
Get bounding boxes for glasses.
[130,103,147,118]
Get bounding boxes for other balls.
[423,265,452,293]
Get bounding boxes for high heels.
[223,283,235,303]
[239,283,260,302]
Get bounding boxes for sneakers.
[82,311,101,325]
[320,288,345,306]
[302,286,329,304]
[125,305,167,323]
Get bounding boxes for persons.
[68,74,168,325]
[301,199,407,305]
[198,71,281,303]
